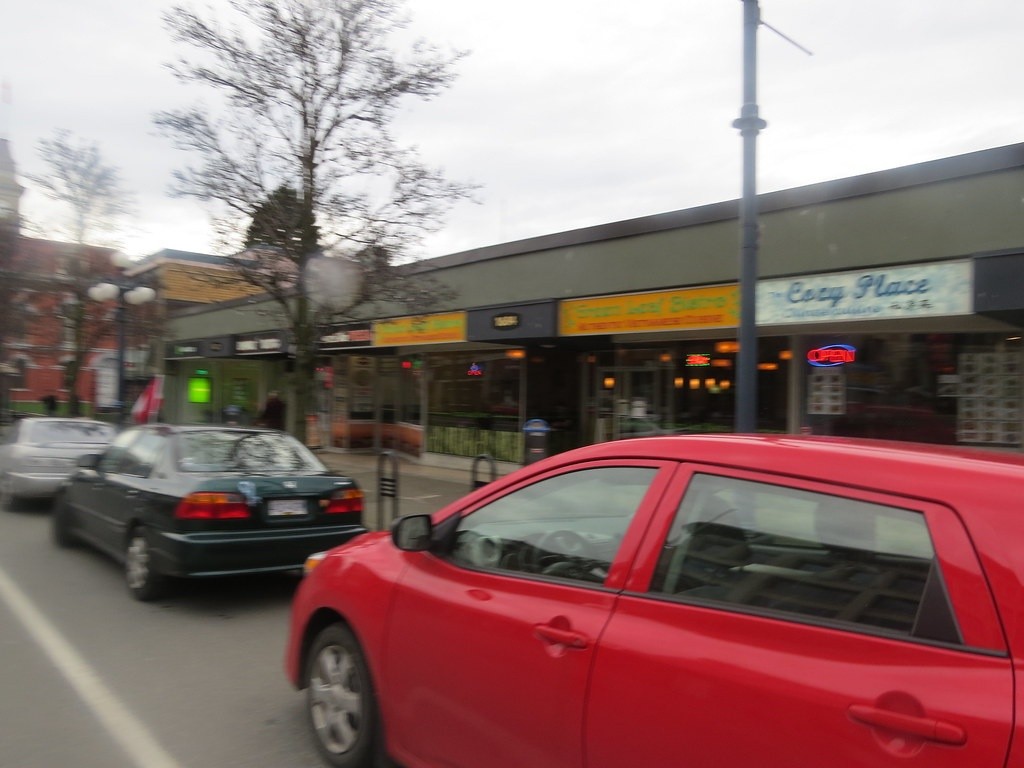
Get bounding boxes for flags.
[131,380,164,423]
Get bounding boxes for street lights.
[86,282,158,422]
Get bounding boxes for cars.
[0,416,120,511]
[56,423,368,602]
[284,432,1024,768]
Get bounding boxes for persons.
[258,393,285,433]
